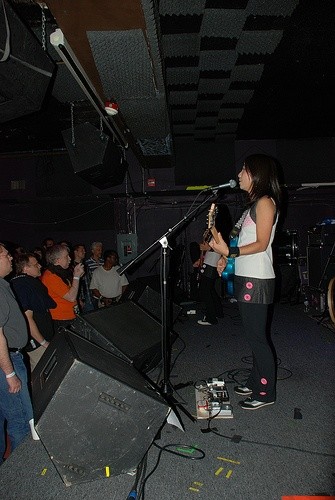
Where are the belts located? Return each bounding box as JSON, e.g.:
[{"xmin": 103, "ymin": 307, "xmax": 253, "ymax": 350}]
[{"xmin": 8, "ymin": 348, "xmax": 20, "ymax": 352}]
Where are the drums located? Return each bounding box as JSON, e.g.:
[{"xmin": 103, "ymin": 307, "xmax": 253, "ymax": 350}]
[{"xmin": 286, "ymin": 226, "xmax": 305, "ymax": 265}]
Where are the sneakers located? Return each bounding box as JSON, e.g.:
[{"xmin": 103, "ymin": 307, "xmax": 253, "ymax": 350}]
[
  {"xmin": 234, "ymin": 387, "xmax": 253, "ymax": 395},
  {"xmin": 197, "ymin": 319, "xmax": 212, "ymax": 326},
  {"xmin": 238, "ymin": 398, "xmax": 274, "ymax": 410}
]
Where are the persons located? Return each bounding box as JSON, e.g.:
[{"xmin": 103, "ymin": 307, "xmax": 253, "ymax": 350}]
[
  {"xmin": 89, "ymin": 250, "xmax": 129, "ymax": 309},
  {"xmin": 30, "ymin": 246, "xmax": 45, "ymax": 263},
  {"xmin": 39, "ymin": 245, "xmax": 85, "ymax": 331},
  {"xmin": 209, "ymin": 154, "xmax": 280, "ymax": 410},
  {"xmin": 0, "ymin": 243, "xmax": 34, "ymax": 463},
  {"xmin": 85, "ymin": 241, "xmax": 104, "ymax": 275},
  {"xmin": 11, "ymin": 254, "xmax": 57, "ymax": 371},
  {"xmin": 197, "ymin": 228, "xmax": 222, "ymax": 325},
  {"xmin": 71, "ymin": 243, "xmax": 91, "ymax": 314},
  {"xmin": 43, "ymin": 239, "xmax": 55, "ymax": 250}
]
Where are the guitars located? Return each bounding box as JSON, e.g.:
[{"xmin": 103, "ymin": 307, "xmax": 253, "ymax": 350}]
[
  {"xmin": 207, "ymin": 203, "xmax": 238, "ymax": 297},
  {"xmin": 198, "ymin": 231, "xmax": 210, "ymax": 268}
]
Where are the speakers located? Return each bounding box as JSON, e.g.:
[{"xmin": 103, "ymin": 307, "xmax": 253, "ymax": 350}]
[
  {"xmin": 69, "ymin": 300, "xmax": 172, "ymax": 362},
  {"xmin": 28, "ymin": 329, "xmax": 171, "ymax": 488},
  {"xmin": 136, "ymin": 283, "xmax": 183, "ymax": 328}
]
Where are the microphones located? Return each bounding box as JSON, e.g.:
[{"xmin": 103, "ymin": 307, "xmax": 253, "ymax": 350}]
[{"xmin": 204, "ymin": 180, "xmax": 236, "ymax": 191}]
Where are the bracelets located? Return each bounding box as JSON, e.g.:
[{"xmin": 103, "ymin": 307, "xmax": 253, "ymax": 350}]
[
  {"xmin": 226, "ymin": 247, "xmax": 239, "ymax": 258},
  {"xmin": 73, "ymin": 277, "xmax": 80, "ymax": 280},
  {"xmin": 5, "ymin": 371, "xmax": 15, "ymax": 378},
  {"xmin": 40, "ymin": 339, "xmax": 46, "ymax": 345}
]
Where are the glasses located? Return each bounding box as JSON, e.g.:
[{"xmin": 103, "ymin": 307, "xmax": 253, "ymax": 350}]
[{"xmin": 1, "ymin": 253, "xmax": 12, "ymax": 258}]
